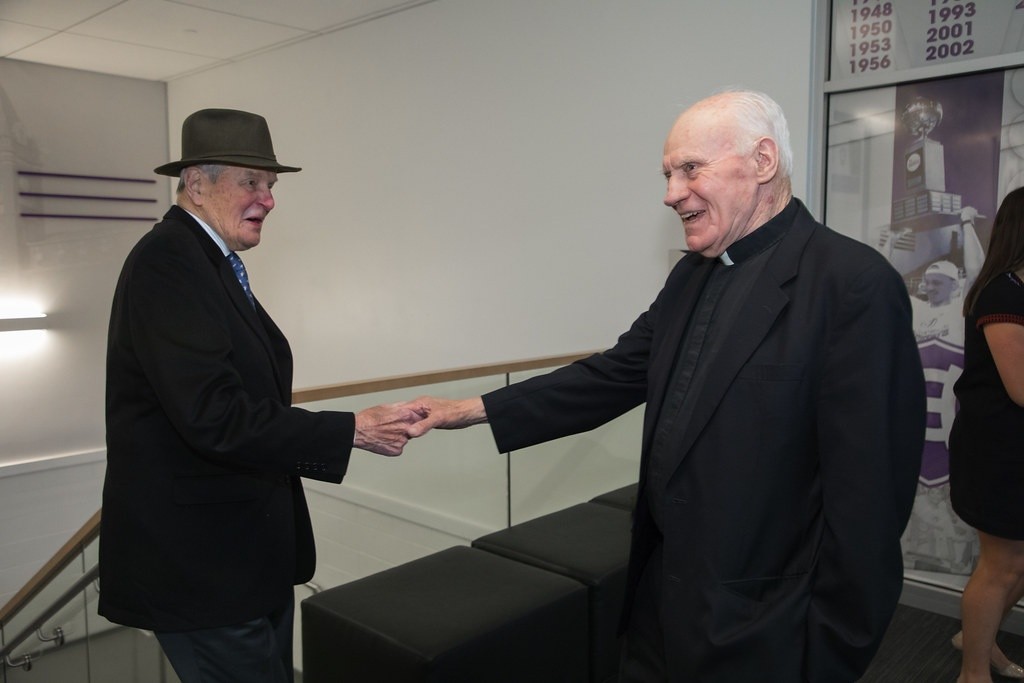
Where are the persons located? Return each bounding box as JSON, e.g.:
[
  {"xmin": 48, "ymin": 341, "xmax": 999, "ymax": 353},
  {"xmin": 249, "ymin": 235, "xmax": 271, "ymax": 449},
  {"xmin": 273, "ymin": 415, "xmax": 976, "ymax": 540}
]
[
  {"xmin": 95, "ymin": 108, "xmax": 424, "ymax": 683},
  {"xmin": 400, "ymin": 90, "xmax": 928, "ymax": 683},
  {"xmin": 949, "ymin": 186, "xmax": 1024, "ymax": 682}
]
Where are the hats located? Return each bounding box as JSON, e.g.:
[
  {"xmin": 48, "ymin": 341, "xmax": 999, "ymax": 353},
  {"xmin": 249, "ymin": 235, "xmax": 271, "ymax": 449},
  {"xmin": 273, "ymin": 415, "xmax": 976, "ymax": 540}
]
[
  {"xmin": 153, "ymin": 108, "xmax": 302, "ymax": 176},
  {"xmin": 926, "ymin": 261, "xmax": 958, "ymax": 281}
]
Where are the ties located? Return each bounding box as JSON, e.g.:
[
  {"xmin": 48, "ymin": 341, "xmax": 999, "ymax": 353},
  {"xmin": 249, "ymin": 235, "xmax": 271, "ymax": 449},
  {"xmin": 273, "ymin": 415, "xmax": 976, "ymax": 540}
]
[{"xmin": 226, "ymin": 251, "xmax": 257, "ymax": 313}]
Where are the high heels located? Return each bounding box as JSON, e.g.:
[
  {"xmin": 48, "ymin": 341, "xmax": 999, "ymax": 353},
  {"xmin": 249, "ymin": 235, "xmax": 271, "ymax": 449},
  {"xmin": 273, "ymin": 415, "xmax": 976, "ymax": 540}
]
[{"xmin": 952, "ymin": 631, "xmax": 1024, "ymax": 678}]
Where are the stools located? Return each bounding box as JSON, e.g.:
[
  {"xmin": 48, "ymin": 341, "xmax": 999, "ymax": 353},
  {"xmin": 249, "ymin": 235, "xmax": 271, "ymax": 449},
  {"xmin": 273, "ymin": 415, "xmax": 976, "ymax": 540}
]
[{"xmin": 300, "ymin": 481, "xmax": 639, "ymax": 683}]
